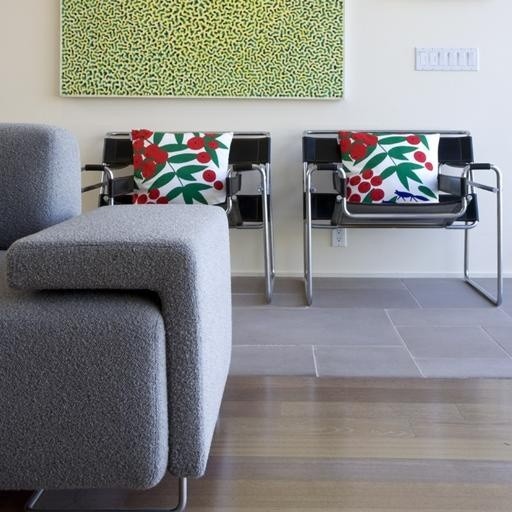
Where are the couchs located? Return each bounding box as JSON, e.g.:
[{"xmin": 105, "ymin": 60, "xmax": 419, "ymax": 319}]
[{"xmin": 0, "ymin": 123, "xmax": 232, "ymax": 512}]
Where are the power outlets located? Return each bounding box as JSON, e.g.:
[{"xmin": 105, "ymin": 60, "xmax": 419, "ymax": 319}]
[{"xmin": 331, "ymin": 228, "xmax": 346, "ymax": 248}]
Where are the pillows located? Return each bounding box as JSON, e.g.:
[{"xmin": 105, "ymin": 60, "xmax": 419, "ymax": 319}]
[
  {"xmin": 129, "ymin": 129, "xmax": 234, "ymax": 205},
  {"xmin": 336, "ymin": 129, "xmax": 439, "ymax": 204}
]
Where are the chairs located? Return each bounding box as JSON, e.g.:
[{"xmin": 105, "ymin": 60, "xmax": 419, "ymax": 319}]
[
  {"xmin": 80, "ymin": 130, "xmax": 275, "ymax": 302},
  {"xmin": 302, "ymin": 129, "xmax": 502, "ymax": 307}
]
